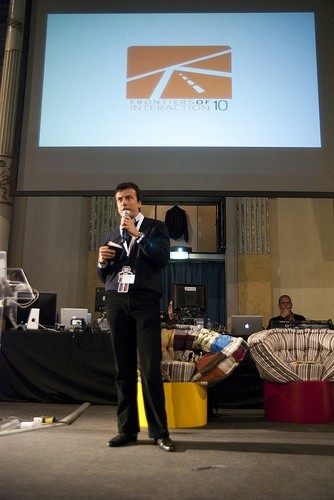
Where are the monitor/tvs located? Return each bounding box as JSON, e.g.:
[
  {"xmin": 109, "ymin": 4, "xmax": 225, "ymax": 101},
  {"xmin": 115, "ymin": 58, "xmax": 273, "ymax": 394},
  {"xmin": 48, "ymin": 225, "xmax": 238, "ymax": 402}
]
[
  {"xmin": 17, "ymin": 291, "xmax": 57, "ymax": 330},
  {"xmin": 95, "ymin": 287, "xmax": 107, "ymax": 312},
  {"xmin": 171, "ymin": 282, "xmax": 208, "ymax": 314}
]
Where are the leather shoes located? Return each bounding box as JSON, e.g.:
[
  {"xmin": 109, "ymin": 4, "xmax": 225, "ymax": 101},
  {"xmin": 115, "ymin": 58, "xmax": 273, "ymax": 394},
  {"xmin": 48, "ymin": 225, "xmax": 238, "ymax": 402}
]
[
  {"xmin": 154, "ymin": 436, "xmax": 176, "ymax": 451},
  {"xmin": 109, "ymin": 433, "xmax": 138, "ymax": 447}
]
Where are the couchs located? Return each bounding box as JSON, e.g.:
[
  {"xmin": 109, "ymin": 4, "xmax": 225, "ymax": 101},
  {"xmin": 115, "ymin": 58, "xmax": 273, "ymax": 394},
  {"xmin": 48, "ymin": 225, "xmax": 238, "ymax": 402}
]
[
  {"xmin": 247, "ymin": 327, "xmax": 334, "ymax": 424},
  {"xmin": 136, "ymin": 325, "xmax": 250, "ymax": 429}
]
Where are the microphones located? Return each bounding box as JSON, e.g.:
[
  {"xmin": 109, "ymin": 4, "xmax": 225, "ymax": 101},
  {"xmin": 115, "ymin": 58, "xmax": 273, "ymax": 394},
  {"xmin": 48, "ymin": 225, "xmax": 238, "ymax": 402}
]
[{"xmin": 121, "ymin": 210, "xmax": 130, "ymax": 243}]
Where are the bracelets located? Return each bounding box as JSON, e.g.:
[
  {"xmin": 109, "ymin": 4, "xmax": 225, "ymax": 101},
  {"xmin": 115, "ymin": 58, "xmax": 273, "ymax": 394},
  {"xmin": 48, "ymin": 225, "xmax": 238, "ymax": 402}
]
[{"xmin": 136, "ymin": 232, "xmax": 144, "ymax": 241}]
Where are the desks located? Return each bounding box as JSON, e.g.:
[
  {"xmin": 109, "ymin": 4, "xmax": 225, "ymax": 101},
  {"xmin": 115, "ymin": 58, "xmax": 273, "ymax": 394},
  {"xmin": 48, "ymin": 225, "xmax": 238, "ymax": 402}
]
[{"xmin": 0, "ymin": 330, "xmax": 264, "ymax": 411}]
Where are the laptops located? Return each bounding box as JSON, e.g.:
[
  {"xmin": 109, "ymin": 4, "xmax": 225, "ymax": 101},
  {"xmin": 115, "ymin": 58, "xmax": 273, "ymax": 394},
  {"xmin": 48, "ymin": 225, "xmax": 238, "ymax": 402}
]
[
  {"xmin": 61, "ymin": 308, "xmax": 88, "ymax": 329},
  {"xmin": 230, "ymin": 315, "xmax": 264, "ymax": 337}
]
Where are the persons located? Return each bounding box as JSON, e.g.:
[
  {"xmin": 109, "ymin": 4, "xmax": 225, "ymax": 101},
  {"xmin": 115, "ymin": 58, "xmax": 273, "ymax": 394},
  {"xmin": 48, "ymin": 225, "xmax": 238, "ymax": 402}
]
[
  {"xmin": 97, "ymin": 181, "xmax": 176, "ymax": 452},
  {"xmin": 266, "ymin": 295, "xmax": 307, "ymax": 330}
]
